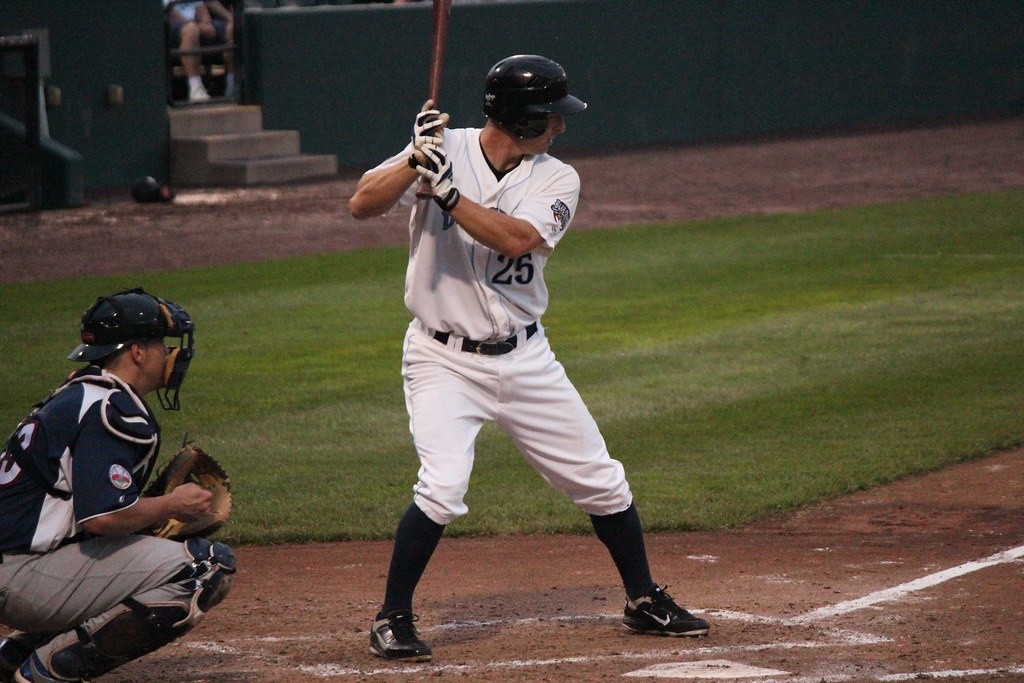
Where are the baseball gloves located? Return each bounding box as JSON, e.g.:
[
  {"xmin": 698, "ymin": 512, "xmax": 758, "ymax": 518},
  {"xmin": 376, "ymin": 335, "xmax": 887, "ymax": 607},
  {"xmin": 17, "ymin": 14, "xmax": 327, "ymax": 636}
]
[{"xmin": 142, "ymin": 431, "xmax": 231, "ymax": 540}]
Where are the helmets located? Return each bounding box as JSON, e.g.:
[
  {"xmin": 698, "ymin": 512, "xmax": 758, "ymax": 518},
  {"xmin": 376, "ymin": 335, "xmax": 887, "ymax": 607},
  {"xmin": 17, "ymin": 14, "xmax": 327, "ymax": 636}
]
[
  {"xmin": 66, "ymin": 288, "xmax": 165, "ymax": 360},
  {"xmin": 483, "ymin": 56, "xmax": 587, "ymax": 139}
]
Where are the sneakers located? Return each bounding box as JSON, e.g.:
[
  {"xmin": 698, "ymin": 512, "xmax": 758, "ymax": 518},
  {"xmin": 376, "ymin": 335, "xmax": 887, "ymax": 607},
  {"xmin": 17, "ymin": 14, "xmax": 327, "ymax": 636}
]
[
  {"xmin": 369, "ymin": 609, "xmax": 432, "ymax": 663},
  {"xmin": 622, "ymin": 582, "xmax": 710, "ymax": 635},
  {"xmin": 14, "ymin": 649, "xmax": 87, "ymax": 683},
  {"xmin": 0, "ymin": 637, "xmax": 32, "ymax": 672}
]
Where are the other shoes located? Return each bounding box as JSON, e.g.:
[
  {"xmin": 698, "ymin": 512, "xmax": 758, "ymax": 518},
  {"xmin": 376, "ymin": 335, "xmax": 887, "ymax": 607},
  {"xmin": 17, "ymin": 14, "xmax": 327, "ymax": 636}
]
[{"xmin": 191, "ymin": 86, "xmax": 212, "ymax": 102}]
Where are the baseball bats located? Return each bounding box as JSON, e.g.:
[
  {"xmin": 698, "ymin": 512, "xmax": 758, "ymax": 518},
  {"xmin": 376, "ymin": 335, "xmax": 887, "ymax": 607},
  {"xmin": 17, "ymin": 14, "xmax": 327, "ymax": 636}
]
[{"xmin": 418, "ymin": 0, "xmax": 452, "ymax": 199}]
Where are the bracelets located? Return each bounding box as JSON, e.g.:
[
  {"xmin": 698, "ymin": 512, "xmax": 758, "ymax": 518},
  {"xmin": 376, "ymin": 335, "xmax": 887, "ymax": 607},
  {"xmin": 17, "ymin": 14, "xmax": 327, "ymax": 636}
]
[{"xmin": 203, "ymin": 19, "xmax": 214, "ymax": 24}]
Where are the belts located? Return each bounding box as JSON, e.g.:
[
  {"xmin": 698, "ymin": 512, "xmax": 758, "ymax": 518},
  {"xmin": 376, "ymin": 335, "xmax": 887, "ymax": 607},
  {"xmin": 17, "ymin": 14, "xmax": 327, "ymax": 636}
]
[{"xmin": 434, "ymin": 321, "xmax": 538, "ymax": 357}]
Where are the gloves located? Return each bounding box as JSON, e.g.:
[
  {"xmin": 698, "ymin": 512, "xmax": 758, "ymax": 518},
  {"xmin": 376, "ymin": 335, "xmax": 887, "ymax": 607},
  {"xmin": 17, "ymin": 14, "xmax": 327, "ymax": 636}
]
[
  {"xmin": 410, "ymin": 140, "xmax": 460, "ymax": 213},
  {"xmin": 407, "ymin": 99, "xmax": 450, "ymax": 169}
]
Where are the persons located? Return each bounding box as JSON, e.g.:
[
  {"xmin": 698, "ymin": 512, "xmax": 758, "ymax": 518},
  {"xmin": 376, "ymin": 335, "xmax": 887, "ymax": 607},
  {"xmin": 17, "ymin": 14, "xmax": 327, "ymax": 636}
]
[
  {"xmin": 161, "ymin": 0, "xmax": 235, "ymax": 103},
  {"xmin": 350, "ymin": 55, "xmax": 711, "ymax": 661},
  {"xmin": 0, "ymin": 288, "xmax": 237, "ymax": 683}
]
